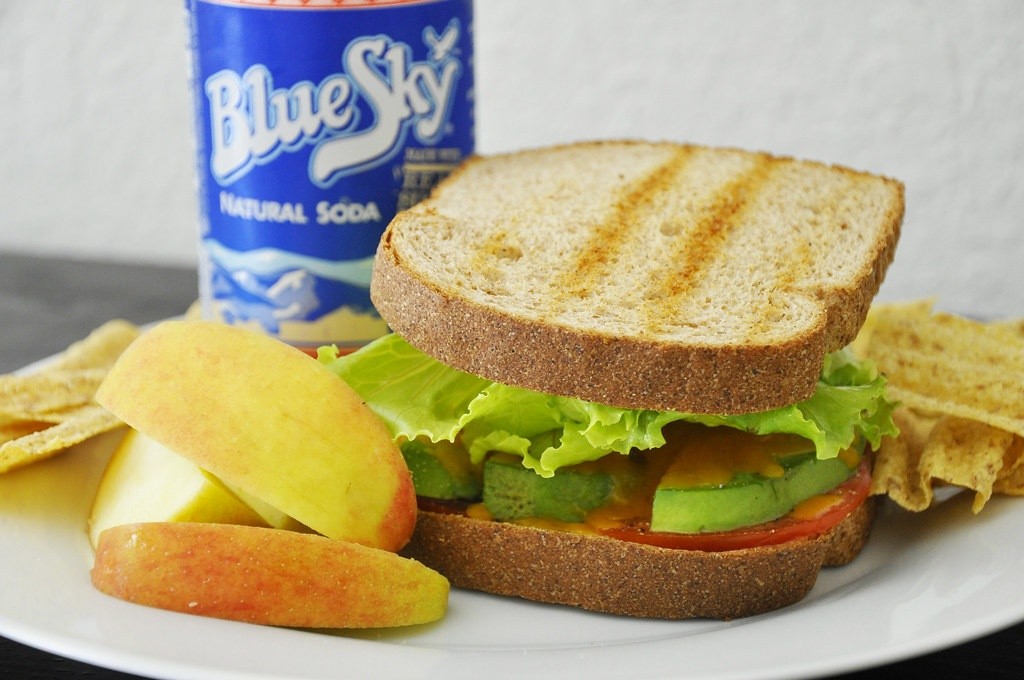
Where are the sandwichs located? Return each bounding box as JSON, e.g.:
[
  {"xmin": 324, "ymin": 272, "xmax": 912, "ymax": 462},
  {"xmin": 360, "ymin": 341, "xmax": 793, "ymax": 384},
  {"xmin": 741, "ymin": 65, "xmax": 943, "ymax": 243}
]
[{"xmin": 306, "ymin": 133, "xmax": 909, "ymax": 622}]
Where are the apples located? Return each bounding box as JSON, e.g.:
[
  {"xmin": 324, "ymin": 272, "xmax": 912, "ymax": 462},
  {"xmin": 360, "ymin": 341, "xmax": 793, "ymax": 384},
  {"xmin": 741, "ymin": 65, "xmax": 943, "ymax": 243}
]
[{"xmin": 88, "ymin": 320, "xmax": 450, "ymax": 628}]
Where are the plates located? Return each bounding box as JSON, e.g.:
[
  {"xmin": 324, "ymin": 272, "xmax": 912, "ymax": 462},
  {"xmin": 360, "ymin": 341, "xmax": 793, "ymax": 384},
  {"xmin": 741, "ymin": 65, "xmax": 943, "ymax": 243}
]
[{"xmin": 8, "ymin": 319, "xmax": 1024, "ymax": 680}]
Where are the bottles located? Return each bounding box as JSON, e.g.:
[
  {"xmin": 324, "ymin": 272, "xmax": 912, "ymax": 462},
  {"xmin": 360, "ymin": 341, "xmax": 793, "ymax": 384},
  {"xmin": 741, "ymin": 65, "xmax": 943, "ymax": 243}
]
[{"xmin": 188, "ymin": 1, "xmax": 482, "ymax": 355}]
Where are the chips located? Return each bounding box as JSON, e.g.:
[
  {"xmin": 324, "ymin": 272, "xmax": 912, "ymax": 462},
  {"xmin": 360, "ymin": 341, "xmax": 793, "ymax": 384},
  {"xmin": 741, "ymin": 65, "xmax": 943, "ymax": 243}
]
[
  {"xmin": 848, "ymin": 299, "xmax": 1024, "ymax": 515},
  {"xmin": 1, "ymin": 315, "xmax": 140, "ymax": 476}
]
